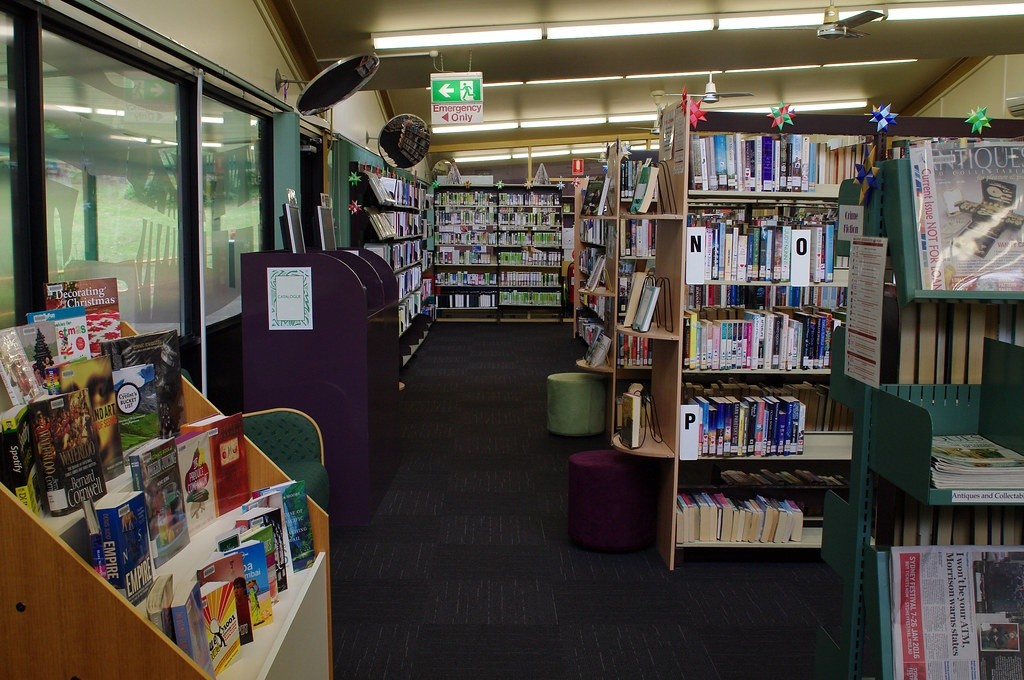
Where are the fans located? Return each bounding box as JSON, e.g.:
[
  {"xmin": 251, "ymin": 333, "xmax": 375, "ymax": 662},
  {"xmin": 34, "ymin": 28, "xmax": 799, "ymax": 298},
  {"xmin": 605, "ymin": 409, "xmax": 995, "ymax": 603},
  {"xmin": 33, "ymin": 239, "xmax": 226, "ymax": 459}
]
[
  {"xmin": 653, "ymin": 73, "xmax": 754, "ymax": 104},
  {"xmin": 625, "ymin": 90, "xmax": 666, "ymax": 135},
  {"xmin": 758, "ymin": 0, "xmax": 885, "ymax": 41}
]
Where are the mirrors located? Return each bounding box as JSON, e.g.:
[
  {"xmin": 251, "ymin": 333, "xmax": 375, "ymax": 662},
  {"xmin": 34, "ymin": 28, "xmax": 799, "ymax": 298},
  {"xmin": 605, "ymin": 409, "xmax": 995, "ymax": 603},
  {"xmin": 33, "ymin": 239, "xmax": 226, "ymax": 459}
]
[
  {"xmin": 275, "ymin": 51, "xmax": 380, "ymax": 116},
  {"xmin": 366, "ymin": 113, "xmax": 431, "ymax": 168}
]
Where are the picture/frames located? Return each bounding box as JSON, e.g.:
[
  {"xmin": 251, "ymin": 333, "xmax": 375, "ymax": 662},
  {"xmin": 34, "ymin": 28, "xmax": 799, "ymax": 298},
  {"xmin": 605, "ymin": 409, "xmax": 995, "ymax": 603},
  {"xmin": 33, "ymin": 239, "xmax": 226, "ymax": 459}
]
[
  {"xmin": 318, "ymin": 206, "xmax": 337, "ymax": 251},
  {"xmin": 286, "ymin": 203, "xmax": 306, "ymax": 254}
]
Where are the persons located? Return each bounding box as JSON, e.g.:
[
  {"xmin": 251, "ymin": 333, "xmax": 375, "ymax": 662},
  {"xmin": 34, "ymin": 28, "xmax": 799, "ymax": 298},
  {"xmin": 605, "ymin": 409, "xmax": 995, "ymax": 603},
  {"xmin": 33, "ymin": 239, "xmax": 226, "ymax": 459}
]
[{"xmin": 232, "ymin": 576, "xmax": 249, "ymax": 626}]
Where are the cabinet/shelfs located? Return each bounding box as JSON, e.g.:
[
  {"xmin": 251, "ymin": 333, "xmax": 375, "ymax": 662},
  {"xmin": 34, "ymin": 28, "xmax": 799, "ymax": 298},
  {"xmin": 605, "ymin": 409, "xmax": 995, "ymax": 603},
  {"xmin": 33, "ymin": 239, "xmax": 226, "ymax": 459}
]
[
  {"xmin": 384, "ymin": 116, "xmax": 430, "ymax": 164},
  {"xmin": 563, "ymin": 95, "xmax": 1024, "ymax": 680},
  {"xmin": 434, "ymin": 185, "xmax": 564, "ymax": 323},
  {"xmin": 348, "ymin": 161, "xmax": 436, "ymax": 371}
]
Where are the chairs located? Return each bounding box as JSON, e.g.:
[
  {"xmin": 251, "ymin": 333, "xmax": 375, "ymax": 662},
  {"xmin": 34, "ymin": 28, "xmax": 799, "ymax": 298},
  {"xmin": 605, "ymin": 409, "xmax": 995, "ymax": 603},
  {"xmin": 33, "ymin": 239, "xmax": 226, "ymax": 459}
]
[{"xmin": 180, "ymin": 368, "xmax": 331, "ymax": 509}]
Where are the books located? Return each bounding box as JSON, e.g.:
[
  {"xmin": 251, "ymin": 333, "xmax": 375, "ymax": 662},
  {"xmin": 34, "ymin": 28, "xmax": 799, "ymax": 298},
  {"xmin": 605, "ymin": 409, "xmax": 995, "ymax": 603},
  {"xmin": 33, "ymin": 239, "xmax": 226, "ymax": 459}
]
[
  {"xmin": 0, "ymin": 276, "xmax": 316, "ymax": 680},
  {"xmin": 367, "ymin": 132, "xmax": 864, "ymax": 542},
  {"xmin": 931, "ymin": 434, "xmax": 1024, "ymax": 489}
]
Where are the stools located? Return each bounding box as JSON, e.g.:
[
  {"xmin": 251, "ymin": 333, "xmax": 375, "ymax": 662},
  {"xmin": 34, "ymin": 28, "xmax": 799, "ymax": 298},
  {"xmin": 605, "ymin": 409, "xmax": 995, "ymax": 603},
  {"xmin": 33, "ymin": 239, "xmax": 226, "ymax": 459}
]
[
  {"xmin": 546, "ymin": 372, "xmax": 606, "ymax": 437},
  {"xmin": 569, "ymin": 450, "xmax": 658, "ymax": 552}
]
